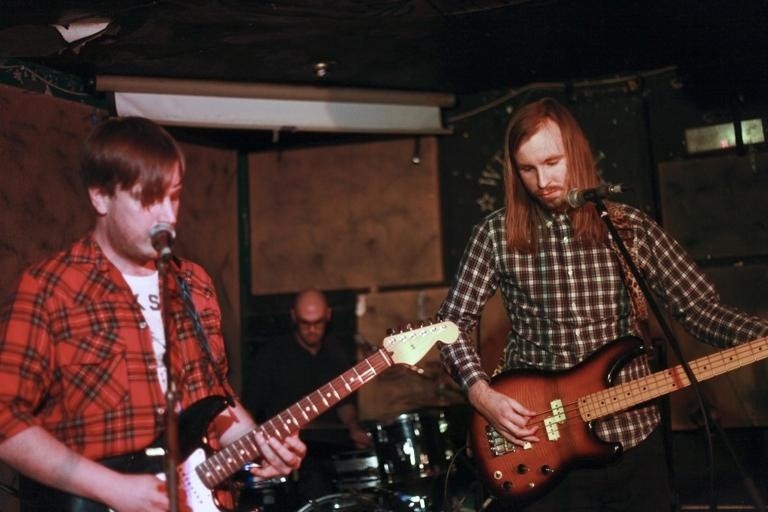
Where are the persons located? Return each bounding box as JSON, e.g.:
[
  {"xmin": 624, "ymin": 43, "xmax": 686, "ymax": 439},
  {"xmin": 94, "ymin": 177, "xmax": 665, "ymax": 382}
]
[
  {"xmin": 433, "ymin": 97, "xmax": 768, "ymax": 511},
  {"xmin": 246, "ymin": 285, "xmax": 373, "ymax": 450},
  {"xmin": 1, "ymin": 115, "xmax": 307, "ymax": 512}
]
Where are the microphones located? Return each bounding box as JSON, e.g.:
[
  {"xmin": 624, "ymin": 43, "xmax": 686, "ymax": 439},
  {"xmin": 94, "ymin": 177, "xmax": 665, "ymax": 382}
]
[
  {"xmin": 149, "ymin": 222, "xmax": 177, "ymax": 264},
  {"xmin": 565, "ymin": 183, "xmax": 624, "ymax": 209}
]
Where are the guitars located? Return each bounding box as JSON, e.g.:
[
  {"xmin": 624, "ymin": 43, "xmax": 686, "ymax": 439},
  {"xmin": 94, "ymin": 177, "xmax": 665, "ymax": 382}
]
[
  {"xmin": 470, "ymin": 334, "xmax": 768, "ymax": 494},
  {"xmin": 106, "ymin": 318, "xmax": 462, "ymax": 512}
]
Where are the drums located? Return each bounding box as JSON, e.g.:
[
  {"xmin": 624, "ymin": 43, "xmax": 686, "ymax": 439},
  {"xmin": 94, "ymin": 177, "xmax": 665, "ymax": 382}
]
[
  {"xmin": 370, "ymin": 409, "xmax": 438, "ymax": 485},
  {"xmin": 332, "ymin": 454, "xmax": 386, "ymax": 488}
]
